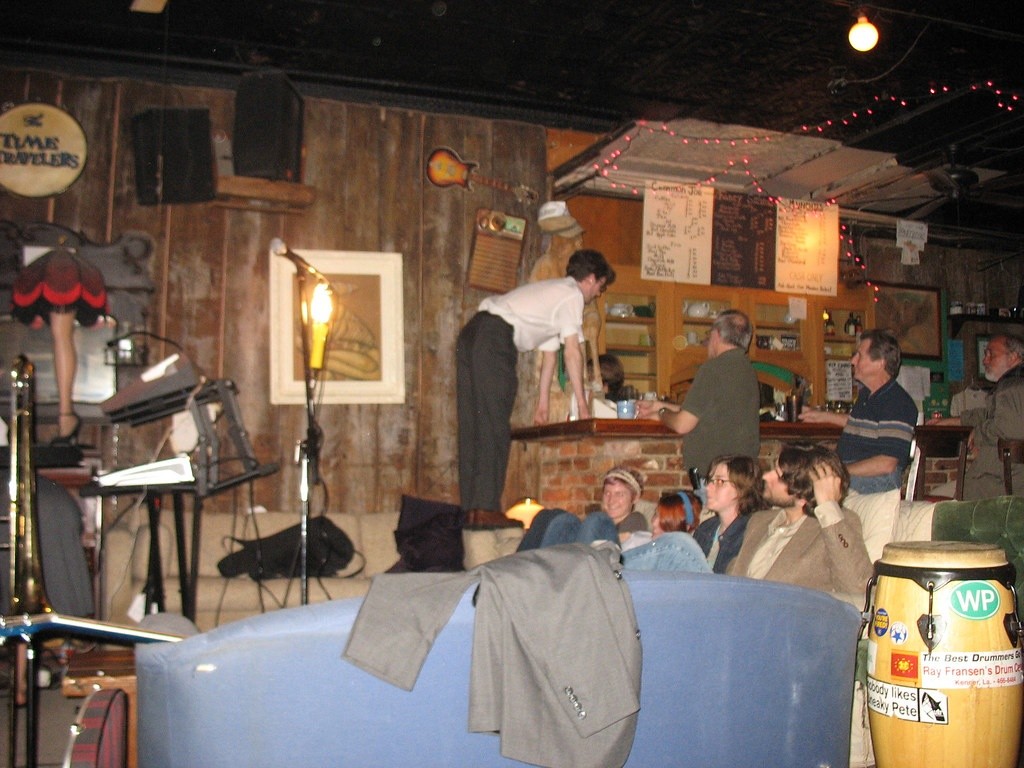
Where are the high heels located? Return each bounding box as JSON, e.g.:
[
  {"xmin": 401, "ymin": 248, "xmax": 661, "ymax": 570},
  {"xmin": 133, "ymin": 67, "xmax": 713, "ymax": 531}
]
[{"xmin": 49, "ymin": 412, "xmax": 82, "ymax": 447}]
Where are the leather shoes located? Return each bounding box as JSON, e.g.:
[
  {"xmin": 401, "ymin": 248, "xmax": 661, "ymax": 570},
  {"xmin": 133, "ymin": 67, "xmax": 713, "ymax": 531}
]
[{"xmin": 465, "ymin": 510, "xmax": 525, "ymax": 531}]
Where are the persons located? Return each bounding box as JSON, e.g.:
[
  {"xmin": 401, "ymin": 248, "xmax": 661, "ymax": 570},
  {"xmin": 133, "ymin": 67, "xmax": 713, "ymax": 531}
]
[
  {"xmin": 782, "ymin": 328, "xmax": 920, "ymax": 566},
  {"xmin": 13, "ymin": 247, "xmax": 107, "ymax": 448},
  {"xmin": 586, "ymin": 354, "xmax": 625, "ymax": 404},
  {"xmin": 635, "ymin": 309, "xmax": 760, "ymax": 502},
  {"xmin": 491, "ymin": 437, "xmax": 873, "ymax": 600},
  {"xmin": 923, "ymin": 334, "xmax": 1024, "ymax": 501},
  {"xmin": 455, "ymin": 249, "xmax": 611, "ymax": 531}
]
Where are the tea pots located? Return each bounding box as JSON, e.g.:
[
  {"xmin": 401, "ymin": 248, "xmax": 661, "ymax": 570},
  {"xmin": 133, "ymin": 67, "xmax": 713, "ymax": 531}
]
[
  {"xmin": 618, "ymin": 385, "xmax": 640, "ymax": 400},
  {"xmin": 683, "ymin": 301, "xmax": 710, "ymax": 317},
  {"xmin": 607, "ymin": 303, "xmax": 633, "ymax": 317}
]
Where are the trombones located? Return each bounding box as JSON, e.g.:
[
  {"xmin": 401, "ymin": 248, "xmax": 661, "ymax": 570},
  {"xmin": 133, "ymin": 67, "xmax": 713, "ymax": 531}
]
[{"xmin": 9, "ymin": 353, "xmax": 58, "ymax": 768}]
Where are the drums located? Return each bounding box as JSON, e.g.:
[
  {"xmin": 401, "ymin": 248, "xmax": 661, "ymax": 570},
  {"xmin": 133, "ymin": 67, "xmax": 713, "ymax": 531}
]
[{"xmin": 866, "ymin": 541, "xmax": 1024, "ymax": 768}]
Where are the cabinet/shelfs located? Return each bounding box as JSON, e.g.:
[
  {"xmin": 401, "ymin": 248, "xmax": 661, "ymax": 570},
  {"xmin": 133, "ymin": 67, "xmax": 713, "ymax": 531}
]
[{"xmin": 585, "ymin": 279, "xmax": 863, "ymax": 411}]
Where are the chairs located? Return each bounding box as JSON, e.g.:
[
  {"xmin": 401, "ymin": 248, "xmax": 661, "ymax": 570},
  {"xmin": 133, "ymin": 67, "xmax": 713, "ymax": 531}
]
[
  {"xmin": 997, "ymin": 437, "xmax": 1024, "ymax": 495},
  {"xmin": 900, "ymin": 439, "xmax": 969, "ymax": 502}
]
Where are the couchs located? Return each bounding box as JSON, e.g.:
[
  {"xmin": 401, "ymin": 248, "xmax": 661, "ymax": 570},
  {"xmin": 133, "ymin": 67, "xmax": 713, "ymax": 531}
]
[{"xmin": 101, "ymin": 487, "xmax": 1024, "ymax": 768}]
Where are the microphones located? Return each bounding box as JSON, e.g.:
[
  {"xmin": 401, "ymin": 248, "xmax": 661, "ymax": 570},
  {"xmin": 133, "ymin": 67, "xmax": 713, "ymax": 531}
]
[{"xmin": 271, "ymin": 238, "xmax": 319, "ymax": 278}]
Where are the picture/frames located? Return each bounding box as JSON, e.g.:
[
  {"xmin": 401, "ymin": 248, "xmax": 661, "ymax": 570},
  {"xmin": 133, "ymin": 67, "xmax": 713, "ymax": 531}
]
[
  {"xmin": 268, "ymin": 248, "xmax": 406, "ymax": 404},
  {"xmin": 864, "ymin": 279, "xmax": 943, "ymax": 362}
]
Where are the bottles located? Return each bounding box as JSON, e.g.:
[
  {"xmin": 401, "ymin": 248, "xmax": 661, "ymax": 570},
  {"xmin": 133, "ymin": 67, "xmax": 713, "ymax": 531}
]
[
  {"xmin": 756, "ymin": 334, "xmax": 800, "ymax": 352},
  {"xmin": 823, "ymin": 306, "xmax": 835, "ymax": 336},
  {"xmin": 844, "ymin": 311, "xmax": 863, "ymax": 348},
  {"xmin": 815, "ymin": 400, "xmax": 855, "ymax": 414},
  {"xmin": 702, "ymin": 331, "xmax": 711, "ymax": 345},
  {"xmin": 949, "ymin": 300, "xmax": 1024, "ymax": 319},
  {"xmin": 104, "ymin": 321, "xmax": 146, "ymax": 365},
  {"xmin": 786, "ymin": 377, "xmax": 803, "ymax": 423}
]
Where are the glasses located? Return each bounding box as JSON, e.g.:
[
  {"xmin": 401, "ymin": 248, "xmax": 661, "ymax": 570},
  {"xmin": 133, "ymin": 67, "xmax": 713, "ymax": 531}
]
[
  {"xmin": 704, "ymin": 322, "xmax": 723, "ymax": 337},
  {"xmin": 595, "ymin": 276, "xmax": 607, "ymax": 293},
  {"xmin": 705, "ymin": 478, "xmax": 734, "ymax": 487}
]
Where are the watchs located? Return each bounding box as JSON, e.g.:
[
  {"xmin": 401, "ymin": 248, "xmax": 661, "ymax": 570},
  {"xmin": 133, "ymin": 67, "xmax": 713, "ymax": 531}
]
[{"xmin": 659, "ymin": 407, "xmax": 670, "ymax": 419}]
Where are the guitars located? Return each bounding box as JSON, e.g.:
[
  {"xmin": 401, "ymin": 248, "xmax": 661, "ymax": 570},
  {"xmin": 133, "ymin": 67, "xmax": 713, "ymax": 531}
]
[{"xmin": 424, "ymin": 146, "xmax": 540, "ymax": 205}]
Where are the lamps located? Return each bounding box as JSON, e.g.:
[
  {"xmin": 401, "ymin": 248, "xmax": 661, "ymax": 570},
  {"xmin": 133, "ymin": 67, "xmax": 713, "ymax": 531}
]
[{"xmin": 8, "ymin": 244, "xmax": 110, "ymax": 469}]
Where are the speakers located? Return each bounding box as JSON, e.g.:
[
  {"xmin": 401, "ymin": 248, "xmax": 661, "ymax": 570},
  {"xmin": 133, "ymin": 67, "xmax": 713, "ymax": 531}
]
[
  {"xmin": 130, "ymin": 105, "xmax": 216, "ymax": 206},
  {"xmin": 230, "ymin": 74, "xmax": 305, "ymax": 184},
  {"xmin": 218, "ymin": 515, "xmax": 355, "ymax": 579}
]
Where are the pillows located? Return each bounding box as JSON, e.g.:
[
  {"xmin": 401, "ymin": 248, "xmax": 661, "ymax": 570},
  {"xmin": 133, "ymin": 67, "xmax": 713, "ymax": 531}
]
[
  {"xmin": 393, "ymin": 512, "xmax": 466, "ymax": 573},
  {"xmin": 840, "ymin": 490, "xmax": 901, "ymax": 567}
]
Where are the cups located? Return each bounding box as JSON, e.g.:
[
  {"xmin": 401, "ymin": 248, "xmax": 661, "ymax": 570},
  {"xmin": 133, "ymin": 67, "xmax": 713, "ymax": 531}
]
[
  {"xmin": 775, "ymin": 403, "xmax": 785, "ymax": 417},
  {"xmin": 686, "ymin": 331, "xmax": 701, "ymax": 345},
  {"xmin": 616, "ymin": 399, "xmax": 640, "ymax": 419}
]
